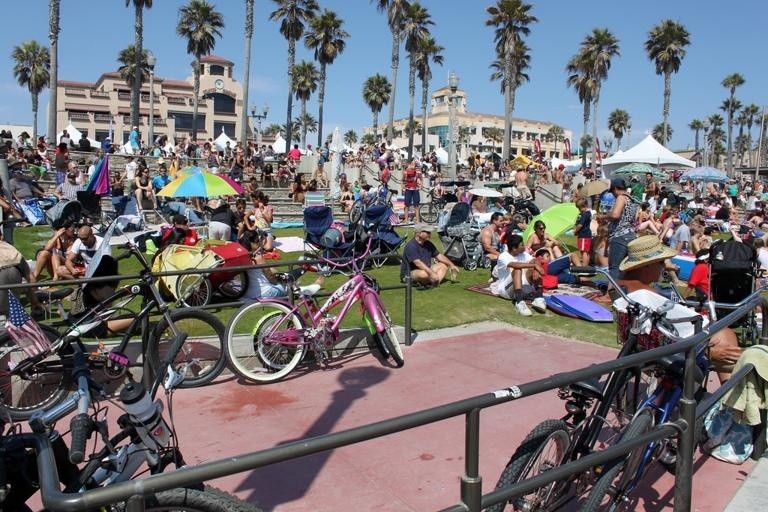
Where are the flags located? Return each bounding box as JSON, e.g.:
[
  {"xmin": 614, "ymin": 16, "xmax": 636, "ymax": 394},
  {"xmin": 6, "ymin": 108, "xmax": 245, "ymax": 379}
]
[{"xmin": 5, "ymin": 288, "xmax": 54, "ymax": 359}]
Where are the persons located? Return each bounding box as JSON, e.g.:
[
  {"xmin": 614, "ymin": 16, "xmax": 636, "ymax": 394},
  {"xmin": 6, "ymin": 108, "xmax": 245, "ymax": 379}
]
[
  {"xmin": 526, "ymin": 249, "xmax": 608, "ymax": 296},
  {"xmin": 287, "ymin": 144, "xmax": 302, "ymax": 161},
  {"xmin": 56, "ymin": 225, "xmax": 111, "ymax": 280},
  {"xmin": 591, "ymin": 178, "xmax": 641, "ymax": 305},
  {"xmin": 0, "ymin": 124, "xmax": 124, "ymax": 228},
  {"xmin": 288, "ymin": 172, "xmax": 310, "ymax": 203},
  {"xmin": 230, "ymin": 200, "xmax": 250, "ymax": 243},
  {"xmin": 304, "ymin": 144, "xmax": 313, "ymax": 157},
  {"xmin": 378, "ymin": 165, "xmax": 391, "ymax": 183},
  {"xmin": 635, "ymin": 175, "xmax": 766, "ymax": 237},
  {"xmin": 420, "ymin": 148, "xmax": 571, "ymax": 223},
  {"xmin": 152, "ymin": 167, "xmax": 174, "ymax": 210},
  {"xmin": 230, "ymin": 165, "xmax": 243, "ymax": 183},
  {"xmin": 128, "ymin": 125, "xmax": 142, "ymax": 156},
  {"xmin": 205, "ymin": 198, "xmax": 236, "ymax": 244},
  {"xmin": 526, "ymin": 220, "xmax": 563, "ymax": 263},
  {"xmin": 139, "ymin": 124, "xmax": 221, "ymax": 170},
  {"xmin": 131, "ymin": 168, "xmax": 158, "ymax": 210},
  {"xmin": 0, "ymin": 227, "xmax": 52, "ymax": 322},
  {"xmin": 473, "ymin": 212, "xmax": 505, "ymax": 261},
  {"xmin": 400, "ymin": 223, "xmax": 460, "ymax": 290},
  {"xmin": 133, "ymin": 157, "xmax": 147, "ymax": 190},
  {"xmin": 313, "ymin": 138, "xmax": 398, "ymax": 169},
  {"xmin": 311, "ymin": 162, "xmax": 328, "ymax": 189},
  {"xmin": 221, "ymin": 127, "xmax": 278, "ymax": 164},
  {"xmin": 277, "ymin": 161, "xmax": 293, "ymax": 181},
  {"xmin": 359, "ymin": 184, "xmax": 373, "ymax": 206},
  {"xmin": 30, "ymin": 218, "xmax": 76, "ymax": 282},
  {"xmin": 571, "ymin": 161, "xmax": 592, "ymax": 203},
  {"xmin": 399, "ymin": 161, "xmax": 424, "ymax": 225},
  {"xmin": 238, "ymin": 230, "xmax": 326, "ymax": 300},
  {"xmin": 150, "ymin": 214, "xmax": 198, "ymax": 266},
  {"xmin": 67, "ymin": 253, "xmax": 142, "ymax": 338},
  {"xmin": 506, "ymin": 214, "xmax": 527, "ymax": 240},
  {"xmin": 351, "ymin": 180, "xmax": 361, "ymax": 193},
  {"xmin": 219, "ymin": 160, "xmax": 232, "ymax": 178},
  {"xmin": 243, "ymin": 176, "xmax": 260, "ymax": 200},
  {"xmin": 119, "ymin": 153, "xmax": 139, "ymax": 198},
  {"xmin": 489, "ymin": 234, "xmax": 548, "ymax": 318},
  {"xmin": 279, "ymin": 153, "xmax": 298, "ymax": 177},
  {"xmin": 340, "ymin": 182, "xmax": 357, "ymax": 215},
  {"xmin": 498, "ymin": 213, "xmax": 514, "ymax": 255},
  {"xmin": 592, "ymin": 235, "xmax": 760, "ymax": 458},
  {"xmin": 261, "ymin": 164, "xmax": 278, "ymax": 188},
  {"xmin": 572, "ymin": 198, "xmax": 592, "ymax": 267},
  {"xmin": 313, "ymin": 172, "xmax": 327, "ymax": 189},
  {"xmin": 244, "ymin": 161, "xmax": 256, "ymax": 178},
  {"xmin": 339, "ymin": 173, "xmax": 349, "ymax": 213},
  {"xmin": 243, "ymin": 189, "xmax": 277, "ymax": 251}
]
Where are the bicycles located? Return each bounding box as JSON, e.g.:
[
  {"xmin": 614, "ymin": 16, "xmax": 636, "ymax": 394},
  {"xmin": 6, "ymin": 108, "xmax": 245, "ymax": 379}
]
[
  {"xmin": 349, "ymin": 188, "xmax": 398, "ymax": 223},
  {"xmin": 420, "ymin": 188, "xmax": 446, "ymax": 222}
]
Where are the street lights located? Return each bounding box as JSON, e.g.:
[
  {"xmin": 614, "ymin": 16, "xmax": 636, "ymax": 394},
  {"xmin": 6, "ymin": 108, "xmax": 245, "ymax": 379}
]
[
  {"xmin": 251, "ymin": 100, "xmax": 269, "ymax": 157},
  {"xmin": 699, "ymin": 116, "xmax": 712, "ymax": 187},
  {"xmin": 442, "ymin": 70, "xmax": 463, "ymax": 182}
]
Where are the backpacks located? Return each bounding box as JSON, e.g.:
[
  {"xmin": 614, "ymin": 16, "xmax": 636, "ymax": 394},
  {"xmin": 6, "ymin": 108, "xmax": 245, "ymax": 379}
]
[
  {"xmin": 70, "ymin": 287, "xmax": 85, "ymax": 316},
  {"xmin": 406, "ymin": 170, "xmax": 417, "ymax": 188}
]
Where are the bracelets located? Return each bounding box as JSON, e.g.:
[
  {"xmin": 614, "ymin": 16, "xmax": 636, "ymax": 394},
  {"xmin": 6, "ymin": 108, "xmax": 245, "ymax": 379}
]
[{"xmin": 262, "ymin": 211, "xmax": 265, "ymax": 215}]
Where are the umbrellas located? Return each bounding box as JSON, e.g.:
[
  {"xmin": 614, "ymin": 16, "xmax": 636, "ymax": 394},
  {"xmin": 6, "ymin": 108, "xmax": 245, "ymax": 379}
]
[
  {"xmin": 462, "ymin": 186, "xmax": 505, "ymax": 207},
  {"xmin": 154, "ymin": 171, "xmax": 246, "ymax": 198},
  {"xmin": 522, "ymin": 202, "xmax": 582, "ymax": 255},
  {"xmin": 608, "ymin": 161, "xmax": 670, "ymax": 182},
  {"xmin": 674, "ymin": 165, "xmax": 733, "ymax": 185},
  {"xmin": 174, "ymin": 166, "xmax": 207, "ymax": 179},
  {"xmin": 573, "ymin": 178, "xmax": 613, "ymax": 212}
]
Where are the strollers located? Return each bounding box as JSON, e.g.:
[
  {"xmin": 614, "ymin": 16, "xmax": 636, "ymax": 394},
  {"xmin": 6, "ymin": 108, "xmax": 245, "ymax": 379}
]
[
  {"xmin": 704, "ymin": 239, "xmax": 760, "ymax": 346},
  {"xmin": 438, "ymin": 203, "xmax": 490, "ymax": 270},
  {"xmin": 35, "ymin": 194, "xmax": 84, "ymax": 265},
  {"xmin": 154, "ymin": 234, "xmax": 251, "ymax": 307}
]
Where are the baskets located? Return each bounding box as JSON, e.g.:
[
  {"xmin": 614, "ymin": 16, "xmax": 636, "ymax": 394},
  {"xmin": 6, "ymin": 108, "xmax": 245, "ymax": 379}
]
[{"xmin": 612, "ymin": 305, "xmax": 678, "ymax": 351}]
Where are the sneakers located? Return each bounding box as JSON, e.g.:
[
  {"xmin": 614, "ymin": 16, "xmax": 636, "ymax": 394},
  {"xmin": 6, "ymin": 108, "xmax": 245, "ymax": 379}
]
[
  {"xmin": 515, "ymin": 301, "xmax": 533, "ymax": 317},
  {"xmin": 531, "ymin": 297, "xmax": 547, "ymax": 313}
]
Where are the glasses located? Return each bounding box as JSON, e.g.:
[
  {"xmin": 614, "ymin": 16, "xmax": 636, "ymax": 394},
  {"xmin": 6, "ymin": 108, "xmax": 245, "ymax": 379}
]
[
  {"xmin": 78, "ymin": 235, "xmax": 90, "ymax": 240},
  {"xmin": 238, "ymin": 206, "xmax": 246, "ymax": 210},
  {"xmin": 73, "ymin": 166, "xmax": 78, "ymax": 169}
]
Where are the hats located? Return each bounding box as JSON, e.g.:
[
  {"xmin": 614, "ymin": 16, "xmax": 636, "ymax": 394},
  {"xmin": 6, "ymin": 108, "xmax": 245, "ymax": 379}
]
[
  {"xmin": 68, "ymin": 172, "xmax": 77, "ymax": 178},
  {"xmin": 7, "ymin": 156, "xmax": 22, "ymax": 167},
  {"xmin": 559, "ymin": 164, "xmax": 566, "ymax": 169},
  {"xmin": 619, "ymin": 235, "xmax": 677, "ymax": 272},
  {"xmin": 124, "ymin": 153, "xmax": 134, "ymax": 159},
  {"xmin": 170, "ymin": 214, "xmax": 187, "ymax": 224},
  {"xmin": 158, "ymin": 157, "xmax": 166, "ymax": 164},
  {"xmin": 414, "ymin": 223, "xmax": 434, "ymax": 233},
  {"xmin": 608, "ymin": 178, "xmax": 624, "ymax": 193},
  {"xmin": 513, "ymin": 213, "xmax": 527, "ymax": 223}
]
